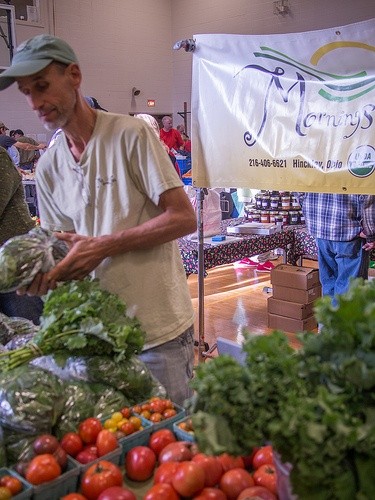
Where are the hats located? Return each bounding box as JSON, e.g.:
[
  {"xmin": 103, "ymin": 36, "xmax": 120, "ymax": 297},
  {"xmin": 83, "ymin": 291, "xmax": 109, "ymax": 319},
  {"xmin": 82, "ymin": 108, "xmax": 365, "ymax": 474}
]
[
  {"xmin": 12, "ymin": 129, "xmax": 23, "ymax": 136},
  {"xmin": 0, "ymin": 123, "xmax": 9, "ymax": 130},
  {"xmin": 1, "ymin": 33, "xmax": 79, "ymax": 91}
]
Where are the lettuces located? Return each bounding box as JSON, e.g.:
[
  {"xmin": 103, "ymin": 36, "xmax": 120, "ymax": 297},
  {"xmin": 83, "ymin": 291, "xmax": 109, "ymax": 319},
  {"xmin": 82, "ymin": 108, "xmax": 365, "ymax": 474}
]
[{"xmin": 185, "ymin": 276, "xmax": 375, "ymax": 500}]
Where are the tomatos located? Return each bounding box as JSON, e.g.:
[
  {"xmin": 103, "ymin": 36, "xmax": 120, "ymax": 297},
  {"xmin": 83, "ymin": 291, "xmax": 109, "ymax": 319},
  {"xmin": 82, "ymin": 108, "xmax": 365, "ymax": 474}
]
[{"xmin": 0, "ymin": 396, "xmax": 278, "ymax": 500}]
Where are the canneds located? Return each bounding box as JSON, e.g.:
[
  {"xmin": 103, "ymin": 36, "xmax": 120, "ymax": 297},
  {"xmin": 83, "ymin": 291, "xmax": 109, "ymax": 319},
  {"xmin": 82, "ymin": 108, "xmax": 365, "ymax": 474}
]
[{"xmin": 243, "ymin": 190, "xmax": 306, "ymax": 225}]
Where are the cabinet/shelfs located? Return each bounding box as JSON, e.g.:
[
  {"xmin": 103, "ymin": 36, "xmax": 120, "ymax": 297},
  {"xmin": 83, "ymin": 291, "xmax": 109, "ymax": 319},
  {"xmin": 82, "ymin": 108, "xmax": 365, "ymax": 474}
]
[{"xmin": 22, "ymin": 180, "xmax": 35, "ymax": 217}]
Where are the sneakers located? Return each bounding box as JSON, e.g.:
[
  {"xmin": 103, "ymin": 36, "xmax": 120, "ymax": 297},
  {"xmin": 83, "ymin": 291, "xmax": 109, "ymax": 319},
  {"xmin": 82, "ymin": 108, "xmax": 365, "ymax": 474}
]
[
  {"xmin": 256, "ymin": 260, "xmax": 275, "ymax": 272},
  {"xmin": 238, "ymin": 258, "xmax": 259, "ymax": 266}
]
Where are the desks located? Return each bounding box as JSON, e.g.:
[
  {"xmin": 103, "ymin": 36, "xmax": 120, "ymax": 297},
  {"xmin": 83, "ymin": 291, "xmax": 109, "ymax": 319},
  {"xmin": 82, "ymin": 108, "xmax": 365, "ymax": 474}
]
[{"xmin": 177, "ymin": 218, "xmax": 318, "ymax": 278}]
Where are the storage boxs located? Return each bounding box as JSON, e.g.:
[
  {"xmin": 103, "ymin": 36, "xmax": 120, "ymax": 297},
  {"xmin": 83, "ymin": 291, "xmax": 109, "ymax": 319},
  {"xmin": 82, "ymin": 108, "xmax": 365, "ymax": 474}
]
[
  {"xmin": 0, "ymin": 397, "xmax": 198, "ymax": 500},
  {"xmin": 177, "ymin": 159, "xmax": 192, "ymax": 185},
  {"xmin": 266, "ymin": 263, "xmax": 321, "ymax": 333}
]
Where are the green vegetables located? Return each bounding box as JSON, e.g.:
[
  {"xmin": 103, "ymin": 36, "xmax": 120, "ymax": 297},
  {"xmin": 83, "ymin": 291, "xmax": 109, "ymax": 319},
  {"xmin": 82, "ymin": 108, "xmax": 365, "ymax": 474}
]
[{"xmin": 0, "ymin": 228, "xmax": 157, "ymax": 467}]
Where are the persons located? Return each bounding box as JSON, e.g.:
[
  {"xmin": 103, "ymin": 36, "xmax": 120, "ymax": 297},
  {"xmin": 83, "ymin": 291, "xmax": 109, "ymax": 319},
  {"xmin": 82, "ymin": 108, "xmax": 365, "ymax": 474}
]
[
  {"xmin": 0, "ymin": 34, "xmax": 197, "ymax": 407},
  {"xmin": 292, "ymin": 192, "xmax": 375, "ymax": 333},
  {"xmin": 0, "ymin": 96, "xmax": 279, "ymax": 324}
]
